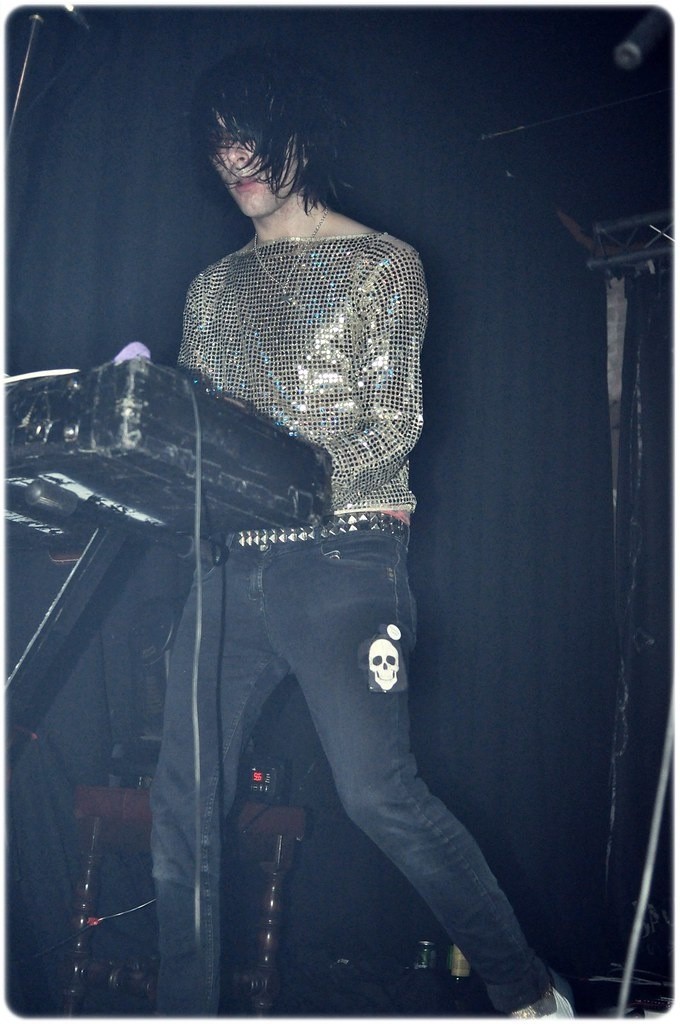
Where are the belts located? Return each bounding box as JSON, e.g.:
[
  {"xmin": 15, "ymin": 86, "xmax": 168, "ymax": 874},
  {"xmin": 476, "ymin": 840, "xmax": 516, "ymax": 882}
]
[{"xmin": 230, "ymin": 499, "xmax": 409, "ymax": 545}]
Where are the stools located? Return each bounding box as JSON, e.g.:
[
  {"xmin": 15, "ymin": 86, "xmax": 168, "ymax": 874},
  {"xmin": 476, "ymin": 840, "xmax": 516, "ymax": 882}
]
[{"xmin": 60, "ymin": 783, "xmax": 307, "ymax": 1016}]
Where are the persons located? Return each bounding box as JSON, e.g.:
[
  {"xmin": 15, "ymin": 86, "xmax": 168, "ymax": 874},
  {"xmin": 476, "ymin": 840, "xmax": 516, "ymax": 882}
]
[{"xmin": 149, "ymin": 48, "xmax": 574, "ymax": 1019}]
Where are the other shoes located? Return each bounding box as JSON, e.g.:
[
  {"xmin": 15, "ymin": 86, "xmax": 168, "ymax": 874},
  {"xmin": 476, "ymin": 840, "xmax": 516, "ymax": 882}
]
[{"xmin": 509, "ymin": 965, "xmax": 574, "ymax": 1019}]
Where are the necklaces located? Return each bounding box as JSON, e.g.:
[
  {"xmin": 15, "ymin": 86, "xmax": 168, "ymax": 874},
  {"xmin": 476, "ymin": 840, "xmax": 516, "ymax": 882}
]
[{"xmin": 254, "ymin": 200, "xmax": 328, "ymax": 305}]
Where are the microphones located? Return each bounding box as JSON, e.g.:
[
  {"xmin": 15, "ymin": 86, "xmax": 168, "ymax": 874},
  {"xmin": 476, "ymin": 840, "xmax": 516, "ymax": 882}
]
[{"xmin": 62, "ymin": 6, "xmax": 92, "ymax": 32}]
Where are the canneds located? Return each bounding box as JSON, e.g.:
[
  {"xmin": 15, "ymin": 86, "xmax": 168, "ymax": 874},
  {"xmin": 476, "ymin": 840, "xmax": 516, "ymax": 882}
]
[{"xmin": 413, "ymin": 941, "xmax": 437, "ymax": 972}]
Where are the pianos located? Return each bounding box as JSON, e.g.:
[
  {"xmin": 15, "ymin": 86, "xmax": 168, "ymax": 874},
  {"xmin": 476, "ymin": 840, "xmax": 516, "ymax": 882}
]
[{"xmin": 5, "ymin": 367, "xmax": 339, "ymax": 545}]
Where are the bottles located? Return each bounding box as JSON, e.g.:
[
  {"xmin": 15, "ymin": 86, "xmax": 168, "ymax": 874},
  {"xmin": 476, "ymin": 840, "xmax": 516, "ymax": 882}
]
[{"xmin": 448, "ymin": 941, "xmax": 471, "ymax": 983}]
